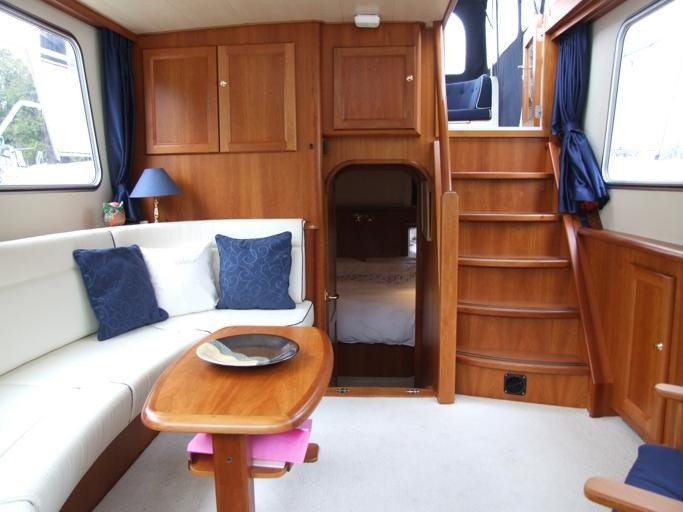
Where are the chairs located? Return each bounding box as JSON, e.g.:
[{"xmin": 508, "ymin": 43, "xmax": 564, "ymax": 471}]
[{"xmin": 584, "ymin": 383, "xmax": 682, "ymax": 512}]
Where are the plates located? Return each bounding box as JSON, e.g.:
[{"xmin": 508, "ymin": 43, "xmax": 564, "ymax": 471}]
[{"xmin": 195, "ymin": 333, "xmax": 300, "ymax": 367}]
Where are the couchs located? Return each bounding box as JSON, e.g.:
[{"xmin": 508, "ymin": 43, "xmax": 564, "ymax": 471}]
[{"xmin": 0, "ymin": 218, "xmax": 314, "ymax": 512}]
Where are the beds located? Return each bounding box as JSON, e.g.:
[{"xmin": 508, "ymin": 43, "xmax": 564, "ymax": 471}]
[{"xmin": 336, "ymin": 256, "xmax": 416, "ymax": 378}]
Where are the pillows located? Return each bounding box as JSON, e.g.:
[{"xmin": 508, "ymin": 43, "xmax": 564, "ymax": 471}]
[{"xmin": 73, "ymin": 230, "xmax": 295, "ymax": 341}]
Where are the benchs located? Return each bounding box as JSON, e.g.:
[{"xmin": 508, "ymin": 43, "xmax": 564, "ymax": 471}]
[{"xmin": 448, "ymin": 74, "xmax": 493, "ymax": 120}]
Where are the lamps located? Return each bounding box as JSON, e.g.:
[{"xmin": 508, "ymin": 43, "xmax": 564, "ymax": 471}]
[{"xmin": 130, "ymin": 167, "xmax": 183, "ymax": 222}]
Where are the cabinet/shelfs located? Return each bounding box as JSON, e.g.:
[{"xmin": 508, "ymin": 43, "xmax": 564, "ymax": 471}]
[
  {"xmin": 142, "ymin": 41, "xmax": 299, "ymax": 156},
  {"xmin": 334, "ymin": 46, "xmax": 417, "ymax": 129}
]
